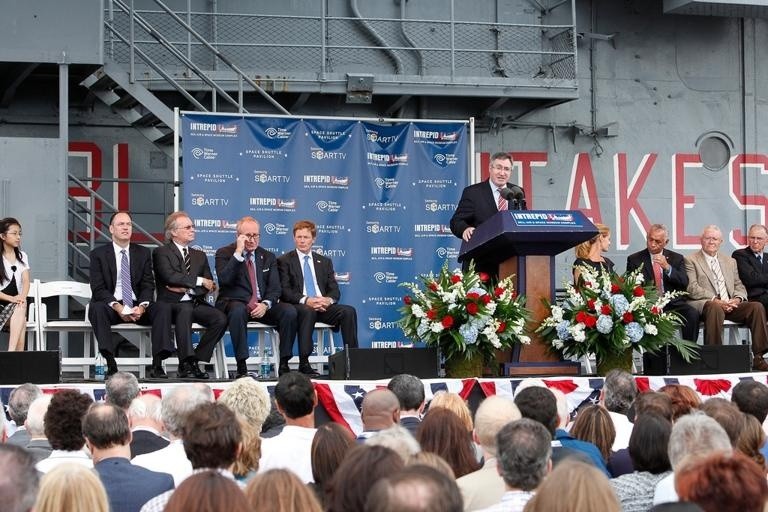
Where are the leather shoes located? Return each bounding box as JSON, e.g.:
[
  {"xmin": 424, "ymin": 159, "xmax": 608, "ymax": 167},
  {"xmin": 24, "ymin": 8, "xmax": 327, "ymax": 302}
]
[
  {"xmin": 177, "ymin": 360, "xmax": 209, "ymax": 378},
  {"xmin": 236, "ymin": 366, "xmax": 247, "ymax": 379},
  {"xmin": 151, "ymin": 368, "xmax": 167, "ymax": 378},
  {"xmin": 299, "ymin": 364, "xmax": 320, "ymax": 376},
  {"xmin": 106, "ymin": 370, "xmax": 117, "ymax": 378},
  {"xmin": 753, "ymin": 358, "xmax": 768, "ymax": 370},
  {"xmin": 279, "ymin": 368, "xmax": 290, "ymax": 377}
]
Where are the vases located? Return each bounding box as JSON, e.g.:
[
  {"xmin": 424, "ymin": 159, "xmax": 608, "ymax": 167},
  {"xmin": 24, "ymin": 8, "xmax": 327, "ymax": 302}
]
[
  {"xmin": 446, "ymin": 352, "xmax": 482, "ymax": 378},
  {"xmin": 596, "ymin": 346, "xmax": 633, "ymax": 377}
]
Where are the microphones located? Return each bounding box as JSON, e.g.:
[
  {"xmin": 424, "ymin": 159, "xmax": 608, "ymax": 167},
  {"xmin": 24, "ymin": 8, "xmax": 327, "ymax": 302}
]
[
  {"xmin": 499, "ymin": 185, "xmax": 519, "ymax": 210},
  {"xmin": 511, "ymin": 187, "xmax": 527, "ymax": 209}
]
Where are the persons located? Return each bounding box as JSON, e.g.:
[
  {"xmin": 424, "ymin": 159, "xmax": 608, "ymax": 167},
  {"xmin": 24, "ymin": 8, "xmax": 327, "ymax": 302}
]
[
  {"xmin": 626, "ymin": 223, "xmax": 701, "ymax": 344},
  {"xmin": 277, "ymin": 220, "xmax": 359, "ymax": 377},
  {"xmin": 0, "ymin": 217, "xmax": 31, "ymax": 351},
  {"xmin": 449, "ymin": 152, "xmax": 528, "ymax": 289},
  {"xmin": 682, "ymin": 225, "xmax": 768, "ymax": 371},
  {"xmin": 151, "ymin": 211, "xmax": 228, "ymax": 379},
  {"xmin": 731, "ymin": 224, "xmax": 768, "ymax": 331},
  {"xmin": 572, "ymin": 221, "xmax": 616, "ymax": 288},
  {"xmin": 214, "ymin": 215, "xmax": 298, "ymax": 378},
  {"xmin": 88, "ymin": 211, "xmax": 175, "ymax": 379},
  {"xmin": 0, "ymin": 366, "xmax": 768, "ymax": 512}
]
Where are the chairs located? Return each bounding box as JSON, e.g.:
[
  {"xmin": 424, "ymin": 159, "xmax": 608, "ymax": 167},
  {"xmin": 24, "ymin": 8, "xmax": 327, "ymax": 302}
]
[{"xmin": 556, "ymin": 288, "xmax": 768, "ymax": 373}]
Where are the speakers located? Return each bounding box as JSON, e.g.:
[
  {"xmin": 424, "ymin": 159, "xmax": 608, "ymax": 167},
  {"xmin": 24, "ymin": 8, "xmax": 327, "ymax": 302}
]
[
  {"xmin": 643, "ymin": 345, "xmax": 754, "ymax": 374},
  {"xmin": 326, "ymin": 347, "xmax": 438, "ymax": 380},
  {"xmin": 0, "ymin": 350, "xmax": 62, "ymax": 384}
]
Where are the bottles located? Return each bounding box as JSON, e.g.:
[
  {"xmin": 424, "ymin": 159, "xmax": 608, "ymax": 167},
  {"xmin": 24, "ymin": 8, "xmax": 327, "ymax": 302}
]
[
  {"xmin": 94, "ymin": 350, "xmax": 106, "ymax": 381},
  {"xmin": 260, "ymin": 347, "xmax": 271, "ymax": 379}
]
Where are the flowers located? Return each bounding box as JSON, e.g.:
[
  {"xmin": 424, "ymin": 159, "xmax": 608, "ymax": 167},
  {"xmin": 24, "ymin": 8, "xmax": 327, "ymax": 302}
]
[
  {"xmin": 534, "ymin": 260, "xmax": 703, "ymax": 368},
  {"xmin": 392, "ymin": 258, "xmax": 541, "ymax": 375}
]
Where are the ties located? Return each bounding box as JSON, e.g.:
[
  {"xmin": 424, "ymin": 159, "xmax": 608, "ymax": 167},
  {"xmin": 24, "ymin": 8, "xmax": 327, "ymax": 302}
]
[
  {"xmin": 497, "ymin": 188, "xmax": 507, "ymax": 211},
  {"xmin": 653, "ymin": 262, "xmax": 662, "ymax": 292},
  {"xmin": 182, "ymin": 248, "xmax": 199, "ymax": 308},
  {"xmin": 121, "ymin": 250, "xmax": 133, "ymax": 309},
  {"xmin": 710, "ymin": 258, "xmax": 730, "ymax": 301},
  {"xmin": 245, "ymin": 253, "xmax": 259, "ymax": 311},
  {"xmin": 303, "ymin": 256, "xmax": 317, "ymax": 299}
]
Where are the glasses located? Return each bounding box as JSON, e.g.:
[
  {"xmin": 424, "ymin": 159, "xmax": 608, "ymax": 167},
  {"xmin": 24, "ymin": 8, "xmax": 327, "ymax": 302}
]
[
  {"xmin": 247, "ymin": 234, "xmax": 260, "ymax": 238},
  {"xmin": 175, "ymin": 224, "xmax": 195, "ymax": 230},
  {"xmin": 6, "ymin": 232, "xmax": 21, "ymax": 235}
]
